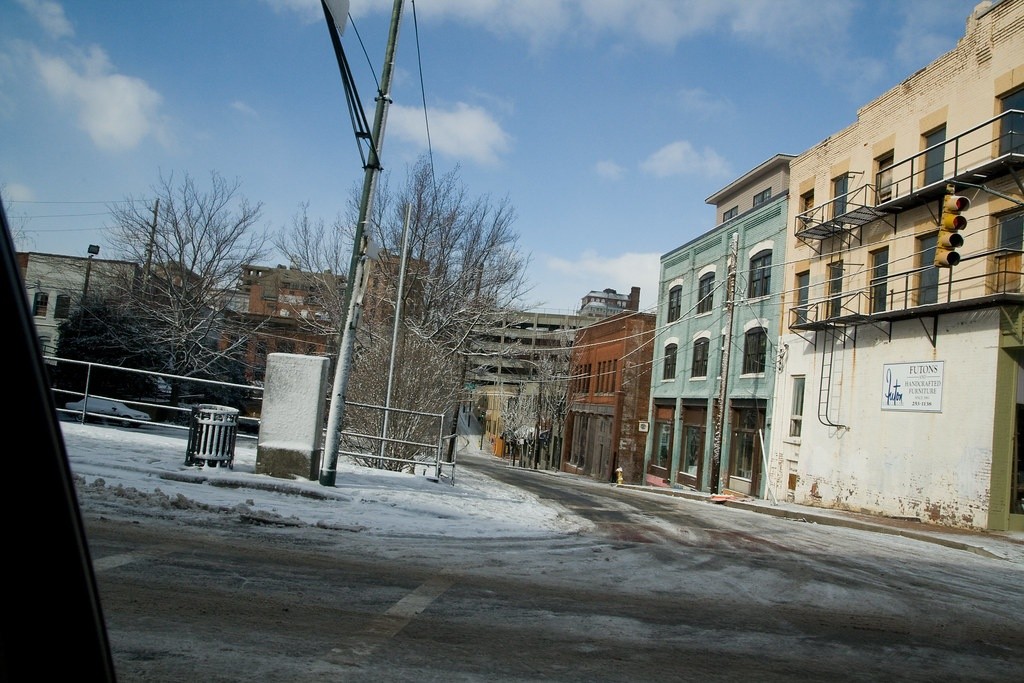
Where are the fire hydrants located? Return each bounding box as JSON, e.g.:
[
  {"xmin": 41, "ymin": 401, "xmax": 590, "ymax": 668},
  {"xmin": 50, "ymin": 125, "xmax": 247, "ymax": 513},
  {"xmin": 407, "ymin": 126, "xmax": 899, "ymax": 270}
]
[{"xmin": 617, "ymin": 467, "xmax": 623, "ymax": 484}]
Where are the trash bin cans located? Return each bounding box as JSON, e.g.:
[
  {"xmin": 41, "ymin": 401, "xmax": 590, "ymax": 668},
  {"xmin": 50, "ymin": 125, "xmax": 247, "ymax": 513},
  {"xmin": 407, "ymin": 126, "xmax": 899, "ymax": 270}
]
[{"xmin": 184, "ymin": 403, "xmax": 242, "ymax": 470}]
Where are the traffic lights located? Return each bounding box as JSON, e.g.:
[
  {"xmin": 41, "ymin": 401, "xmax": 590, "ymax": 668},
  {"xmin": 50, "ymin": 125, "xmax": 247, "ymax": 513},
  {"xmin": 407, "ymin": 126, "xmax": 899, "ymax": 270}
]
[{"xmin": 935, "ymin": 195, "xmax": 971, "ymax": 268}]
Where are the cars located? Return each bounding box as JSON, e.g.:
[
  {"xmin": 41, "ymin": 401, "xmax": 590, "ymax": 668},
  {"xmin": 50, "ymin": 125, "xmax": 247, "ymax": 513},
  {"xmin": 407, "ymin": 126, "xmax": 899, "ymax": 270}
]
[{"xmin": 65, "ymin": 397, "xmax": 152, "ymax": 428}]
